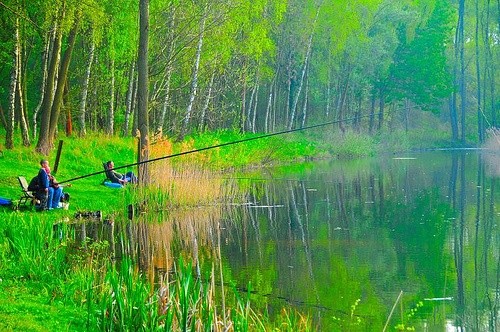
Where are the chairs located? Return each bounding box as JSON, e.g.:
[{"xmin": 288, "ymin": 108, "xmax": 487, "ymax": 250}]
[
  {"xmin": 101, "ymin": 162, "xmax": 128, "ymax": 187},
  {"xmin": 17, "ymin": 176, "xmax": 46, "ymax": 211}
]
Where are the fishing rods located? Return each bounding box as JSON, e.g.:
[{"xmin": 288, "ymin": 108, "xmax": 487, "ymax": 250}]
[
  {"xmin": 49, "ymin": 99, "xmax": 445, "ymax": 190},
  {"xmin": 60, "ymin": 236, "xmax": 379, "ymax": 322}
]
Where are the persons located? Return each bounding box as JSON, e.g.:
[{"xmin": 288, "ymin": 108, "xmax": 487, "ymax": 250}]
[
  {"xmin": 107, "ymin": 161, "xmax": 138, "ymax": 183},
  {"xmin": 45, "ymin": 167, "xmax": 62, "ymax": 209},
  {"xmin": 39, "ymin": 160, "xmax": 49, "ymax": 211}
]
